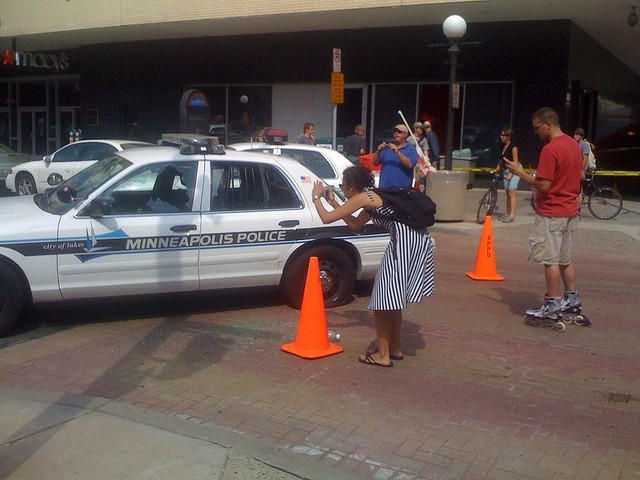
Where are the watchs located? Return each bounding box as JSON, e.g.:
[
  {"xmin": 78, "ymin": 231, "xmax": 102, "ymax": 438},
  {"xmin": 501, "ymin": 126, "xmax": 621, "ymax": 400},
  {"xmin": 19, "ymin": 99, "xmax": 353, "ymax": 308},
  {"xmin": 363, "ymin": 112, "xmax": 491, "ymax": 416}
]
[{"xmin": 312, "ymin": 195, "xmax": 320, "ymax": 202}]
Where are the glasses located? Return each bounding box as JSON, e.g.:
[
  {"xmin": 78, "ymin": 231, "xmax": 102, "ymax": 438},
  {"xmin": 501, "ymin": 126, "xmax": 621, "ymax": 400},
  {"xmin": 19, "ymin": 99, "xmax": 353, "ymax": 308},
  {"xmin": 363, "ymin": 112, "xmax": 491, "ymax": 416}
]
[
  {"xmin": 534, "ymin": 123, "xmax": 546, "ymax": 130},
  {"xmin": 339, "ymin": 184, "xmax": 343, "ymax": 188},
  {"xmin": 393, "ymin": 130, "xmax": 402, "ymax": 133},
  {"xmin": 501, "ymin": 134, "xmax": 507, "ymax": 137}
]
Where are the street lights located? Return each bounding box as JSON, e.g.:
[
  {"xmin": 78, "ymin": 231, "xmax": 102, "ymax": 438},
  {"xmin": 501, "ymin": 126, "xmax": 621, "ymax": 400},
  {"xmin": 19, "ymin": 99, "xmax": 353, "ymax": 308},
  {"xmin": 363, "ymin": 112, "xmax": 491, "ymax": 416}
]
[
  {"xmin": 425, "ymin": 14, "xmax": 469, "ymax": 222},
  {"xmin": 240, "ymin": 95, "xmax": 249, "ymax": 137}
]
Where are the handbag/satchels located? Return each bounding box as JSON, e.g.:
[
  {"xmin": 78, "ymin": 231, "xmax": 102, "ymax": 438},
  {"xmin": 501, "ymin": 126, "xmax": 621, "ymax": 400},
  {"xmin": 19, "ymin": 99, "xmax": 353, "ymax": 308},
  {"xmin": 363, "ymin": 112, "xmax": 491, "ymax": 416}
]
[{"xmin": 364, "ymin": 187, "xmax": 436, "ymax": 229}]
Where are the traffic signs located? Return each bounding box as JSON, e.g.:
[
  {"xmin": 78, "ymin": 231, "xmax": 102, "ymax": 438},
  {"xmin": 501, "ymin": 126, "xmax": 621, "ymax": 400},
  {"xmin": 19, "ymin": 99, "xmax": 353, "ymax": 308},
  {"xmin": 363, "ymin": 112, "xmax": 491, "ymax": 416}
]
[{"xmin": 452, "ymin": 83, "xmax": 461, "ymax": 110}]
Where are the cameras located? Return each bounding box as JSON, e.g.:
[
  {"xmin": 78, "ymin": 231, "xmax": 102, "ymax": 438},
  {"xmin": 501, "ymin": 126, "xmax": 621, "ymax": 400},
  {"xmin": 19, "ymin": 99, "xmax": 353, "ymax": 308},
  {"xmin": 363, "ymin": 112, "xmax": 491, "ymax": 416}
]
[
  {"xmin": 386, "ymin": 143, "xmax": 390, "ymax": 148},
  {"xmin": 323, "ymin": 187, "xmax": 329, "ymax": 198}
]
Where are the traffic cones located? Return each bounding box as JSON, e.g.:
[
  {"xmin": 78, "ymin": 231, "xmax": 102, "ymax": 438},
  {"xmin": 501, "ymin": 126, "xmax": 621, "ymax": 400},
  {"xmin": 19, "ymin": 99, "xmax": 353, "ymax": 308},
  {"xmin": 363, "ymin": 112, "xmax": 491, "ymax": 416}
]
[
  {"xmin": 464, "ymin": 214, "xmax": 505, "ymax": 282},
  {"xmin": 280, "ymin": 255, "xmax": 345, "ymax": 358}
]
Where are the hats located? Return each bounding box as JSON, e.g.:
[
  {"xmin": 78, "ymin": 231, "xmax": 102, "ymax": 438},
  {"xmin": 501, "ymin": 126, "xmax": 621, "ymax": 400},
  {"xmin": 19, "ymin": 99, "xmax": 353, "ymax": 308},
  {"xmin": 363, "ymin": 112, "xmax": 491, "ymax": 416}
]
[
  {"xmin": 575, "ymin": 129, "xmax": 585, "ymax": 135},
  {"xmin": 394, "ymin": 125, "xmax": 407, "ymax": 131},
  {"xmin": 413, "ymin": 122, "xmax": 424, "ymax": 128}
]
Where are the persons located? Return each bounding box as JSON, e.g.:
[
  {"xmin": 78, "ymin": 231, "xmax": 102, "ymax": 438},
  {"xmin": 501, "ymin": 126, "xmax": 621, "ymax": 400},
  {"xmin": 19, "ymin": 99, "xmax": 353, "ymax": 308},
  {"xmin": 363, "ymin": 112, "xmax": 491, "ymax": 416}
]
[
  {"xmin": 312, "ymin": 166, "xmax": 435, "ymax": 366},
  {"xmin": 503, "ymin": 107, "xmax": 592, "ymax": 332},
  {"xmin": 575, "ymin": 128, "xmax": 597, "ymax": 216},
  {"xmin": 372, "ymin": 125, "xmax": 419, "ymax": 192},
  {"xmin": 423, "ymin": 122, "xmax": 441, "ymax": 169},
  {"xmin": 343, "ymin": 125, "xmax": 367, "ymax": 159},
  {"xmin": 297, "ymin": 124, "xmax": 316, "ymax": 144},
  {"xmin": 490, "ymin": 128, "xmax": 523, "ymax": 223},
  {"xmin": 407, "ymin": 122, "xmax": 428, "ymax": 156}
]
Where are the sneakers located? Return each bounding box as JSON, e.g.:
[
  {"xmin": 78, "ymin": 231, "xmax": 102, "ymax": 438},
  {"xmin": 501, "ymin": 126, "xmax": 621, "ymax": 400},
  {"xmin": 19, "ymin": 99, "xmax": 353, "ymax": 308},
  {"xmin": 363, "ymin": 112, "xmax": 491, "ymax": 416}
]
[
  {"xmin": 498, "ymin": 214, "xmax": 508, "ymax": 221},
  {"xmin": 502, "ymin": 216, "xmax": 513, "ymax": 223}
]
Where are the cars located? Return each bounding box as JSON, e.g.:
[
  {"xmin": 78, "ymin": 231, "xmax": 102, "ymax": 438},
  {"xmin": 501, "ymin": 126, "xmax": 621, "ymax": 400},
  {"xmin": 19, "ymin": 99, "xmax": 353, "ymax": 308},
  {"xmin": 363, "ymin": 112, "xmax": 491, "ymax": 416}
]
[
  {"xmin": 4, "ymin": 138, "xmax": 160, "ymax": 197},
  {"xmin": 0, "ymin": 132, "xmax": 434, "ymax": 337},
  {"xmin": 225, "ymin": 127, "xmax": 382, "ymax": 201}
]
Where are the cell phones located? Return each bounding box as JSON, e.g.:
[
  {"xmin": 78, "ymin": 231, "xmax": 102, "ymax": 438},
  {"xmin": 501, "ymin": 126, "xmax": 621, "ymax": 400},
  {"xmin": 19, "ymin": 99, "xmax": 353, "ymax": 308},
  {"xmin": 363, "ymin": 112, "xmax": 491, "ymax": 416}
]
[{"xmin": 501, "ymin": 152, "xmax": 510, "ymax": 162}]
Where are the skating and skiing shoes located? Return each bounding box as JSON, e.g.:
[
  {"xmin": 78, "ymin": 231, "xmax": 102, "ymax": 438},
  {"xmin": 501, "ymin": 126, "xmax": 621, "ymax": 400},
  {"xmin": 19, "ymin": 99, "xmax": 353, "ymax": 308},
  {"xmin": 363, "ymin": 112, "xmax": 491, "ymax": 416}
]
[
  {"xmin": 561, "ymin": 292, "xmax": 591, "ymax": 326},
  {"xmin": 524, "ymin": 293, "xmax": 565, "ymax": 332}
]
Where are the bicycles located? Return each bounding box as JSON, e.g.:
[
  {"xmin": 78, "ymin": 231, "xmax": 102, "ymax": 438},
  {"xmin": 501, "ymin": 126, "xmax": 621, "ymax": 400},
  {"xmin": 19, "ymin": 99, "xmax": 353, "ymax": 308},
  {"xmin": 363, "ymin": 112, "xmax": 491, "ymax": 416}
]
[
  {"xmin": 531, "ymin": 168, "xmax": 623, "ymax": 219},
  {"xmin": 475, "ymin": 170, "xmax": 507, "ymax": 225}
]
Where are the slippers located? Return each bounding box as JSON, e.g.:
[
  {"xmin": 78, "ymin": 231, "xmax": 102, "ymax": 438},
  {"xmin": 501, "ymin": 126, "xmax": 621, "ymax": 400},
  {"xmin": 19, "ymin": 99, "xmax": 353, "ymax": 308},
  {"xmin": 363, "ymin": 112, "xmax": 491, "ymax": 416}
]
[
  {"xmin": 359, "ymin": 354, "xmax": 393, "ymax": 367},
  {"xmin": 368, "ymin": 348, "xmax": 403, "ymax": 360}
]
